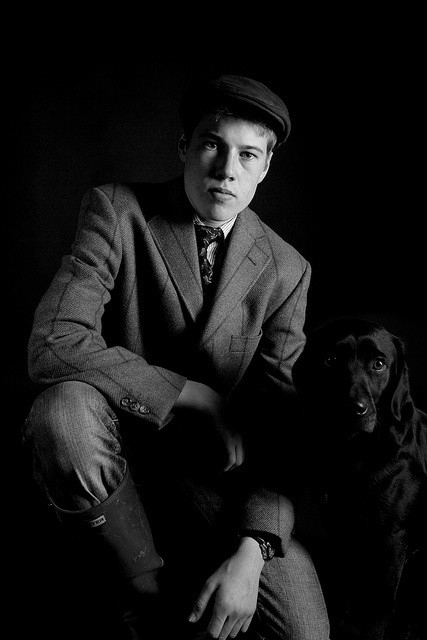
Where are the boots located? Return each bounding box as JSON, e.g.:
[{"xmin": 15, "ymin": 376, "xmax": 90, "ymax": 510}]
[{"xmin": 45, "ymin": 457, "xmax": 164, "ymax": 640}]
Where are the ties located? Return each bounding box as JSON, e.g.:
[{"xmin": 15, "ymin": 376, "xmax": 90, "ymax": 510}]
[{"xmin": 194, "ymin": 224, "xmax": 224, "ymax": 286}]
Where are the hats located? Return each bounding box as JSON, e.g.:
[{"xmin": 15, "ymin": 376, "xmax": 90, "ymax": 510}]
[{"xmin": 180, "ymin": 75, "xmax": 292, "ymax": 152}]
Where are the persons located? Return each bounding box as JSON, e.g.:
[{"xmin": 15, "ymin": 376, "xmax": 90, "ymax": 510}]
[{"xmin": 15, "ymin": 73, "xmax": 333, "ymax": 639}]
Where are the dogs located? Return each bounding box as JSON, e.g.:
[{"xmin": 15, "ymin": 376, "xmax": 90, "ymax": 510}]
[{"xmin": 305, "ymin": 327, "xmax": 426, "ymax": 640}]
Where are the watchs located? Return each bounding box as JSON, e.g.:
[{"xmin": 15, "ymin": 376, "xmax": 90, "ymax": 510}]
[{"xmin": 250, "ymin": 532, "xmax": 278, "ymax": 561}]
